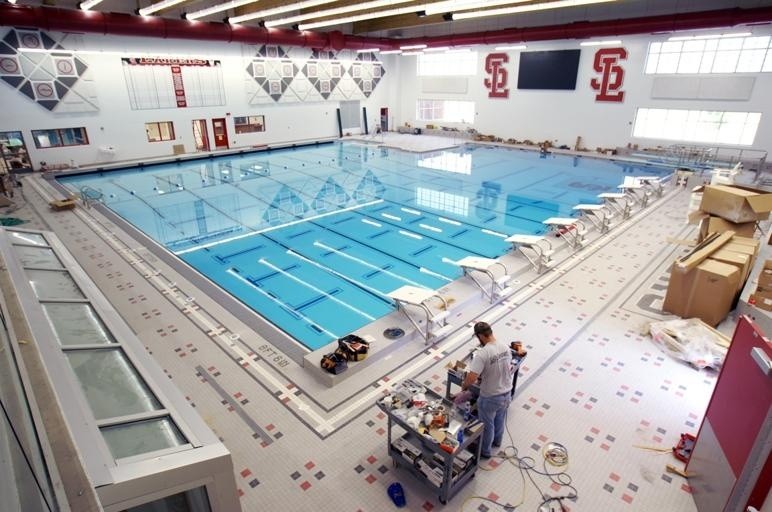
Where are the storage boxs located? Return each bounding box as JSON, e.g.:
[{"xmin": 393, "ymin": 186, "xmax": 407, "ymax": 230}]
[{"xmin": 662, "ymin": 183, "xmax": 772, "ymax": 328}]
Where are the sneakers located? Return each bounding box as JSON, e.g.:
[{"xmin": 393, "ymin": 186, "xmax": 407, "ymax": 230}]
[
  {"xmin": 468, "ymin": 443, "xmax": 492, "ymax": 459},
  {"xmin": 476, "ymin": 435, "xmax": 502, "ymax": 447}
]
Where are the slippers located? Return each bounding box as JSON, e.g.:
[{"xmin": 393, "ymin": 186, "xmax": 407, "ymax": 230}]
[{"xmin": 388, "ymin": 482, "xmax": 406, "ymax": 507}]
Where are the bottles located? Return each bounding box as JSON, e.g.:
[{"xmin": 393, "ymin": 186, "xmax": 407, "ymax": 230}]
[
  {"xmin": 458, "ymin": 430, "xmax": 463, "ymax": 443},
  {"xmin": 464, "ymin": 402, "xmax": 470, "ymax": 421},
  {"xmin": 443, "ymin": 411, "xmax": 449, "ymax": 428}
]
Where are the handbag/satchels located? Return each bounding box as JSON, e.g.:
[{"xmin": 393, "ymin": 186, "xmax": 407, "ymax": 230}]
[
  {"xmin": 321, "ymin": 353, "xmax": 348, "ymax": 375},
  {"xmin": 335, "ymin": 334, "xmax": 369, "ymax": 362}
]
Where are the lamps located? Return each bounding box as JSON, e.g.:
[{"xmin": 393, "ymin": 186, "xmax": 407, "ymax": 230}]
[{"xmin": 78, "ymin": 0, "xmax": 621, "ymax": 56}]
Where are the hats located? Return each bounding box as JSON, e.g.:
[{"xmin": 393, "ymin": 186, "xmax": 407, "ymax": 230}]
[{"xmin": 472, "ymin": 322, "xmax": 491, "ymax": 337}]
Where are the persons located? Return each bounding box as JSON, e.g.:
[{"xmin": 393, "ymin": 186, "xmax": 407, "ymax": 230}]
[{"xmin": 462, "ymin": 322, "xmax": 513, "ymax": 458}]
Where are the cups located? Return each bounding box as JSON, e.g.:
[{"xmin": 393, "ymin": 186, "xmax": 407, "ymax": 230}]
[{"xmin": 384, "ymin": 397, "xmax": 392, "ymax": 411}]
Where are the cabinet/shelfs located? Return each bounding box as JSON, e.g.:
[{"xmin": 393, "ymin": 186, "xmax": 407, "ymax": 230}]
[
  {"xmin": 376, "ymin": 380, "xmax": 485, "ymax": 505},
  {"xmin": 446, "ymin": 345, "xmax": 525, "ymax": 416}
]
